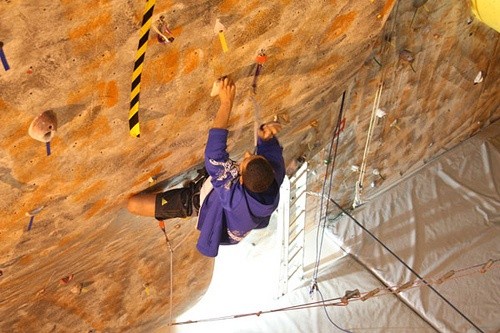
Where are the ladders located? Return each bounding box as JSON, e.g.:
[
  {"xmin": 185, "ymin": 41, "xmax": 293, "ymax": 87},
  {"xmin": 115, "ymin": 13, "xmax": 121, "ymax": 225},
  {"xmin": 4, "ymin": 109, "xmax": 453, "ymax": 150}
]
[{"xmin": 277, "ymin": 158, "xmax": 309, "ymax": 297}]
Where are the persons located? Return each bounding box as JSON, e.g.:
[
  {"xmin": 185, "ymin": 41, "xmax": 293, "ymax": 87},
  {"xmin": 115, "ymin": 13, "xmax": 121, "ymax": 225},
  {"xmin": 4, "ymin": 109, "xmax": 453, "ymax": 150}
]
[{"xmin": 125, "ymin": 76, "xmax": 286, "ymax": 262}]
